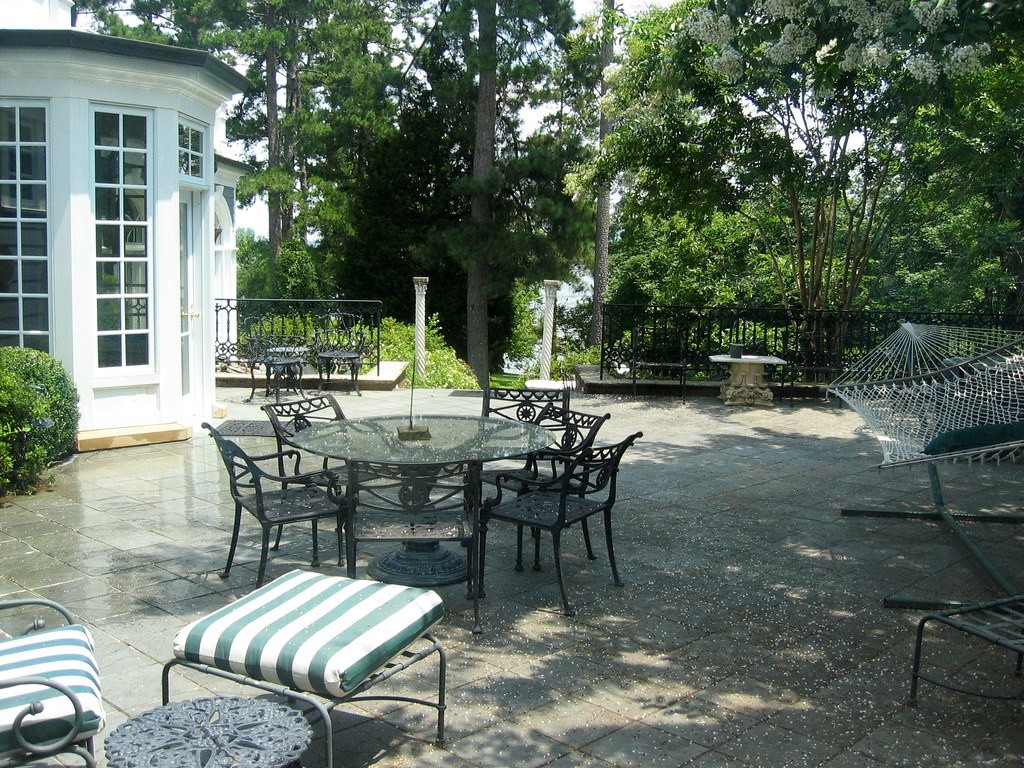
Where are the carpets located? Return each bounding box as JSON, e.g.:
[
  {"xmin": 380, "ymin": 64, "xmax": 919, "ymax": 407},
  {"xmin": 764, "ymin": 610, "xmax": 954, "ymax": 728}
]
[{"xmin": 209, "ymin": 419, "xmax": 327, "ymax": 437}]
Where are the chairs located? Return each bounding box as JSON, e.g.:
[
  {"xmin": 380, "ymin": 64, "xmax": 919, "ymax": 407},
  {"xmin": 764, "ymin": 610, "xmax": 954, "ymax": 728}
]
[
  {"xmin": 463, "ymin": 402, "xmax": 611, "ymax": 572},
  {"xmin": 478, "ymin": 431, "xmax": 644, "ymax": 614},
  {"xmin": 260, "ymin": 394, "xmax": 400, "ymax": 566},
  {"xmin": 201, "ymin": 422, "xmax": 346, "ymax": 590},
  {"xmin": 311, "ymin": 309, "xmax": 374, "ymax": 396},
  {"xmin": 239, "ymin": 313, "xmax": 308, "ymax": 405},
  {"xmin": 632, "ymin": 326, "xmax": 688, "ymax": 405},
  {"xmin": 0, "ymin": 598, "xmax": 108, "ymax": 768},
  {"xmin": 461, "ymin": 387, "xmax": 571, "ymax": 507}
]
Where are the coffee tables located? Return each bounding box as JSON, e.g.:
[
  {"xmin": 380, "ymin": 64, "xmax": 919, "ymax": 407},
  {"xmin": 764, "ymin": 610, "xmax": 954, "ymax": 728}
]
[{"xmin": 103, "ymin": 697, "xmax": 313, "ymax": 768}]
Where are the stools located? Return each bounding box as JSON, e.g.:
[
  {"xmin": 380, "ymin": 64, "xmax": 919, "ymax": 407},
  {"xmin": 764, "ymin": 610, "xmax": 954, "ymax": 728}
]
[{"xmin": 162, "ymin": 569, "xmax": 447, "ymax": 768}]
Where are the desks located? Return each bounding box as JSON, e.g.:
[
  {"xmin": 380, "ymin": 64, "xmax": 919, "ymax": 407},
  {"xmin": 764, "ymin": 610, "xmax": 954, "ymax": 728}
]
[
  {"xmin": 293, "ymin": 415, "xmax": 557, "ymax": 634},
  {"xmin": 708, "ymin": 355, "xmax": 787, "ymax": 407},
  {"xmin": 265, "ymin": 346, "xmax": 312, "ymax": 397}
]
